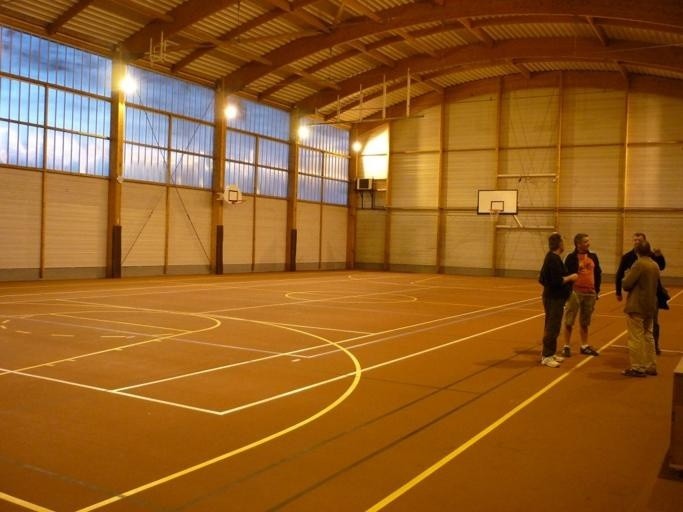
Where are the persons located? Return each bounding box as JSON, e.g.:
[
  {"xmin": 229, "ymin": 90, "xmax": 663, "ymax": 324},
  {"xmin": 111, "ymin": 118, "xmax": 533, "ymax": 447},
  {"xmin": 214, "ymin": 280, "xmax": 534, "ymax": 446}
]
[
  {"xmin": 537, "ymin": 233, "xmax": 579, "ymax": 368},
  {"xmin": 562, "ymin": 233, "xmax": 602, "ymax": 357},
  {"xmin": 622, "ymin": 240, "xmax": 661, "ymax": 377},
  {"xmin": 615, "ymin": 232, "xmax": 670, "ymax": 355}
]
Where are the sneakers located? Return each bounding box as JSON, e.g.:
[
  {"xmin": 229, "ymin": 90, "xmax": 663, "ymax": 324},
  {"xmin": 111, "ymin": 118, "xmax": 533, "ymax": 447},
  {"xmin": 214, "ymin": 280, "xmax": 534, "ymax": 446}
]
[
  {"xmin": 540, "ymin": 354, "xmax": 564, "ymax": 367},
  {"xmin": 622, "ymin": 368, "xmax": 656, "ymax": 377},
  {"xmin": 580, "ymin": 346, "xmax": 599, "ymax": 356},
  {"xmin": 563, "ymin": 347, "xmax": 570, "ymax": 356}
]
